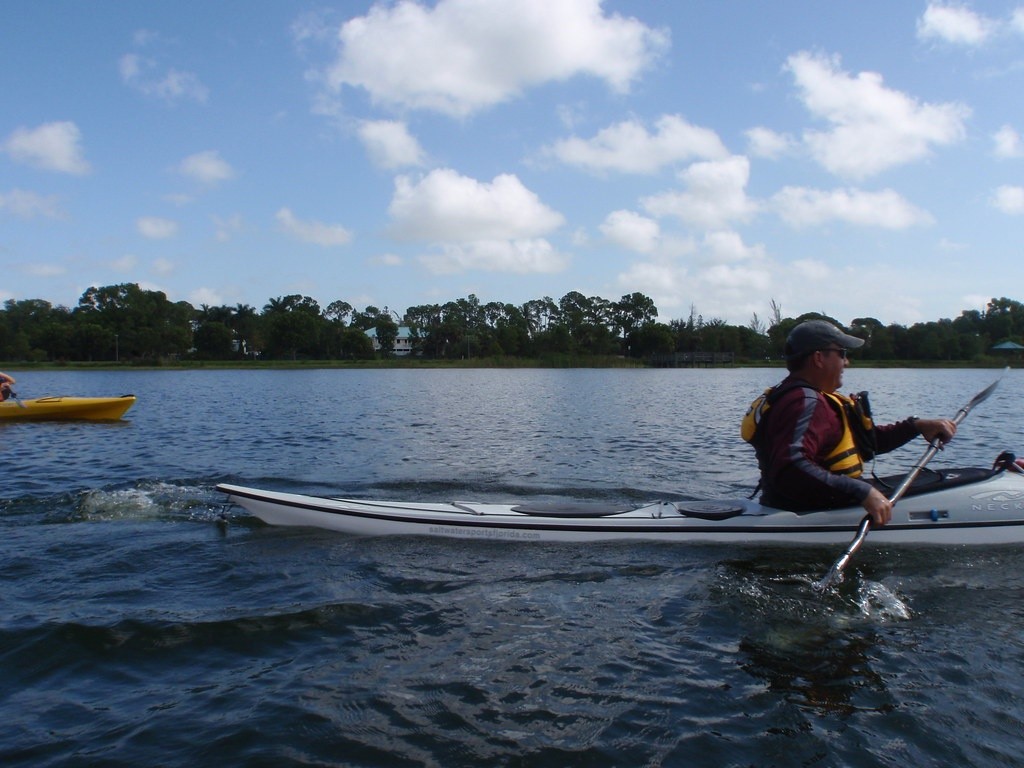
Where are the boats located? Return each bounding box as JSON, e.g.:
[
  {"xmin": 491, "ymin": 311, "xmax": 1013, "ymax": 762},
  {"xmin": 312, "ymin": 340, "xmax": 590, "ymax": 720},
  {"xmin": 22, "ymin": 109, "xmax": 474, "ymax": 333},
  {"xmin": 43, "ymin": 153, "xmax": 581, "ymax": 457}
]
[
  {"xmin": 216, "ymin": 454, "xmax": 1024, "ymax": 545},
  {"xmin": 0, "ymin": 394, "xmax": 136, "ymax": 420}
]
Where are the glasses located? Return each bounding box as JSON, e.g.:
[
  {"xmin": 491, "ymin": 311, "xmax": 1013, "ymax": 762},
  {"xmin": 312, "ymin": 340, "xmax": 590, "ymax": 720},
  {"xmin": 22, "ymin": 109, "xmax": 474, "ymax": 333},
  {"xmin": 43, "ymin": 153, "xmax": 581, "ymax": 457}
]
[{"xmin": 810, "ymin": 349, "xmax": 847, "ymax": 360}]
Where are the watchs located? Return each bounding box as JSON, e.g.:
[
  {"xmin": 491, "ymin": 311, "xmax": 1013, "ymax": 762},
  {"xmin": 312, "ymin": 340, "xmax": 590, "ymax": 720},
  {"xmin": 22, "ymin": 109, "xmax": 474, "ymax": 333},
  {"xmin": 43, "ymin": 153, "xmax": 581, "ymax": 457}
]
[{"xmin": 907, "ymin": 415, "xmax": 920, "ymax": 437}]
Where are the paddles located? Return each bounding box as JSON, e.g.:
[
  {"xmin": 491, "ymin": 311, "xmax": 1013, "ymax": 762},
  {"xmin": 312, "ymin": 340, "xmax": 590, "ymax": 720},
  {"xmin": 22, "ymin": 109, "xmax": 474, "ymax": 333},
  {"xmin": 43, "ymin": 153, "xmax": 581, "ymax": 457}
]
[
  {"xmin": 812, "ymin": 364, "xmax": 1014, "ymax": 588},
  {"xmin": 6, "ymin": 387, "xmax": 27, "ymax": 409}
]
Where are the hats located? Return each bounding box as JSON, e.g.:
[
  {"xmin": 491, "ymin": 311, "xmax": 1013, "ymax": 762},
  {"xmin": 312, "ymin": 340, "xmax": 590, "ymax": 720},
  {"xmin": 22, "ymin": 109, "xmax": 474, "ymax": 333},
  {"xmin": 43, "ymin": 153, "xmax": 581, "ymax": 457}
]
[{"xmin": 784, "ymin": 320, "xmax": 865, "ymax": 356}]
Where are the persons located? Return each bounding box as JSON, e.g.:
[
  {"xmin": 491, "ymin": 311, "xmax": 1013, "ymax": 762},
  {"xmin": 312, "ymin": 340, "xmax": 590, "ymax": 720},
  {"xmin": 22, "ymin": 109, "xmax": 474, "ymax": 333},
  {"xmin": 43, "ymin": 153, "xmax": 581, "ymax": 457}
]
[
  {"xmin": 0, "ymin": 372, "xmax": 16, "ymax": 402},
  {"xmin": 741, "ymin": 320, "xmax": 957, "ymax": 529}
]
